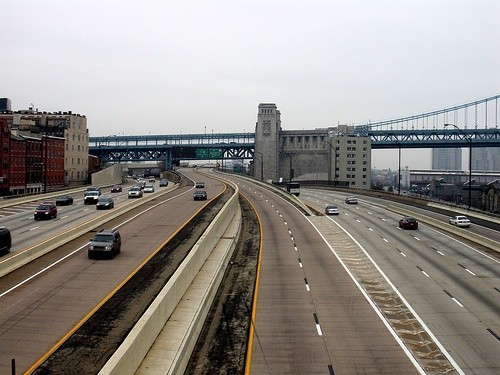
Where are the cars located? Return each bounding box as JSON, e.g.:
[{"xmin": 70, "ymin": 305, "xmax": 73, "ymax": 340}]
[
  {"xmin": 193, "ymin": 190, "xmax": 207, "ymax": 201},
  {"xmin": 143, "ymin": 185, "xmax": 154, "ymax": 193},
  {"xmin": 449, "ymin": 215, "xmax": 471, "ymax": 228},
  {"xmin": 324, "ymin": 205, "xmax": 339, "ymax": 215},
  {"xmin": 110, "ymin": 185, "xmax": 122, "ymax": 193},
  {"xmin": 56, "ymin": 195, "xmax": 74, "ymax": 206},
  {"xmin": 196, "ymin": 181, "xmax": 205, "ymax": 188},
  {"xmin": 345, "ymin": 196, "xmax": 358, "ymax": 204},
  {"xmin": 126, "ymin": 173, "xmax": 169, "ymax": 190},
  {"xmin": 96, "ymin": 197, "xmax": 114, "ymax": 210}
]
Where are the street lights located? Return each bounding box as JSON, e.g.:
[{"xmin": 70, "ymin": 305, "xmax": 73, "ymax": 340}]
[{"xmin": 444, "ymin": 123, "xmax": 472, "ymax": 211}]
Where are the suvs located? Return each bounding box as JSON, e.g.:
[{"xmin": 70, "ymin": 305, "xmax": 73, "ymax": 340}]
[
  {"xmin": 128, "ymin": 186, "xmax": 143, "ymax": 198},
  {"xmin": 0, "ymin": 225, "xmax": 12, "ymax": 256},
  {"xmin": 88, "ymin": 228, "xmax": 122, "ymax": 260},
  {"xmin": 34, "ymin": 201, "xmax": 58, "ymax": 220},
  {"xmin": 399, "ymin": 216, "xmax": 419, "ymax": 230},
  {"xmin": 83, "ymin": 190, "xmax": 100, "ymax": 205},
  {"xmin": 87, "ymin": 187, "xmax": 101, "ymax": 195},
  {"xmin": 285, "ymin": 183, "xmax": 300, "ymax": 196}
]
